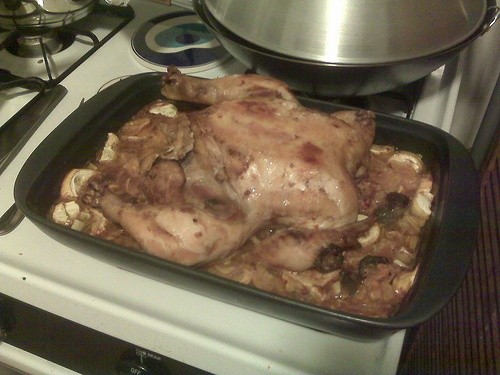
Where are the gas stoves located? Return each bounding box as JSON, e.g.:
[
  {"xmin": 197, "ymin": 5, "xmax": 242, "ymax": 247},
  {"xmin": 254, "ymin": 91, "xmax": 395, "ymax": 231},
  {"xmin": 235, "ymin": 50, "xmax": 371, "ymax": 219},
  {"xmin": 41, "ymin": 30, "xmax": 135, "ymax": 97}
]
[{"xmin": 0, "ymin": 0, "xmax": 471, "ymax": 375}]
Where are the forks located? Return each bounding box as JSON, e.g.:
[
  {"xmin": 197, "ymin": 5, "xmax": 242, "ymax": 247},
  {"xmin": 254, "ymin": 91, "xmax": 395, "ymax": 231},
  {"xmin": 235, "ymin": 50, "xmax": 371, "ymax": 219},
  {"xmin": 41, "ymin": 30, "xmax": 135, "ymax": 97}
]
[{"xmin": 0, "ymin": 97, "xmax": 86, "ymax": 237}]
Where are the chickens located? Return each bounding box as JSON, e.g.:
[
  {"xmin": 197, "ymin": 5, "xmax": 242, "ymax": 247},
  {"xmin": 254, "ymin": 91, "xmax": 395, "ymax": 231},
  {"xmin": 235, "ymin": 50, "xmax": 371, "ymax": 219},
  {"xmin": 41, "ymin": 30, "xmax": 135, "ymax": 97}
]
[{"xmin": 83, "ymin": 64, "xmax": 420, "ymax": 273}]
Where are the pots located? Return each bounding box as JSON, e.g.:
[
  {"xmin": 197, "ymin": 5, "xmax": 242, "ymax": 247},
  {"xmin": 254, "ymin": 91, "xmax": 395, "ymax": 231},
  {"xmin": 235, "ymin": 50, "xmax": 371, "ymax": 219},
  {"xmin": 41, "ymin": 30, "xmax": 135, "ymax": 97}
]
[{"xmin": 155, "ymin": 0, "xmax": 500, "ymax": 101}]
